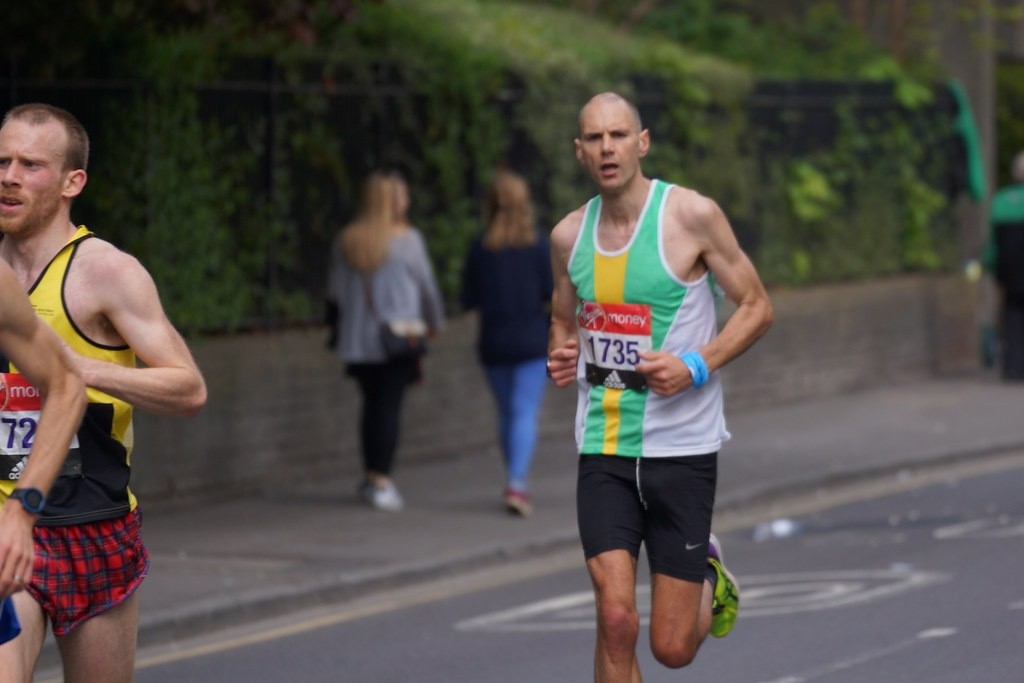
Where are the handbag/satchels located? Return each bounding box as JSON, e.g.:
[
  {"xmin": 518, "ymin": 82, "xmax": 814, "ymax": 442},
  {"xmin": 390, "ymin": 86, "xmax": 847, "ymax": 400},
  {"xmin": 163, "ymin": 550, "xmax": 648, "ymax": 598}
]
[{"xmin": 381, "ymin": 319, "xmax": 428, "ymax": 357}]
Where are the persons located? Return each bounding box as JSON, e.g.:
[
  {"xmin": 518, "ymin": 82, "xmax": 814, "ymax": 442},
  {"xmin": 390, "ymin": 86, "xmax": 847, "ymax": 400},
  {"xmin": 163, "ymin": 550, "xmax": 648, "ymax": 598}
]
[
  {"xmin": 547, "ymin": 92, "xmax": 775, "ymax": 683},
  {"xmin": 459, "ymin": 170, "xmax": 559, "ymax": 517},
  {"xmin": 323, "ymin": 168, "xmax": 447, "ymax": 515},
  {"xmin": 0, "ymin": 102, "xmax": 208, "ymax": 683}
]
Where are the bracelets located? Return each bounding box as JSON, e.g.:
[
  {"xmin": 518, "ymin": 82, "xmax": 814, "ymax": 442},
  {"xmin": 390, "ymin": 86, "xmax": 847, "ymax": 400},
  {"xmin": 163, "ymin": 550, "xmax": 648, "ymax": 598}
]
[
  {"xmin": 680, "ymin": 351, "xmax": 709, "ymax": 388},
  {"xmin": 546, "ymin": 360, "xmax": 551, "ymax": 377}
]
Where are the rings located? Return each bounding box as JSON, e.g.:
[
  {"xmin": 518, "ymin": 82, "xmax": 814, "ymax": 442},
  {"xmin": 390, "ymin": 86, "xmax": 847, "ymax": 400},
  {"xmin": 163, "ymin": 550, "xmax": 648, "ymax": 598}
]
[{"xmin": 14, "ymin": 576, "xmax": 23, "ymax": 581}]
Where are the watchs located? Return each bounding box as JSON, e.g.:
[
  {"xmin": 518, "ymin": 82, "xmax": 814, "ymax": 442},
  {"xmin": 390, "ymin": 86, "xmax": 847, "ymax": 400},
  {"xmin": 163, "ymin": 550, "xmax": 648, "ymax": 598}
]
[{"xmin": 9, "ymin": 487, "xmax": 47, "ymax": 514}]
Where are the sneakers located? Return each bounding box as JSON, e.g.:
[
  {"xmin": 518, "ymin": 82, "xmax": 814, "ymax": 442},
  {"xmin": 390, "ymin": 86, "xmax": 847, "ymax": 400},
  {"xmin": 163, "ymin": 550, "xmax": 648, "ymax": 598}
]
[{"xmin": 706, "ymin": 533, "xmax": 739, "ymax": 639}]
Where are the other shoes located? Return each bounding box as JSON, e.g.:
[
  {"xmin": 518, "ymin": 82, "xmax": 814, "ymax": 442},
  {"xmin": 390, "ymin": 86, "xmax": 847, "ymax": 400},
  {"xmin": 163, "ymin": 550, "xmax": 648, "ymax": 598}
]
[
  {"xmin": 362, "ymin": 480, "xmax": 404, "ymax": 511},
  {"xmin": 503, "ymin": 489, "xmax": 531, "ymax": 516}
]
